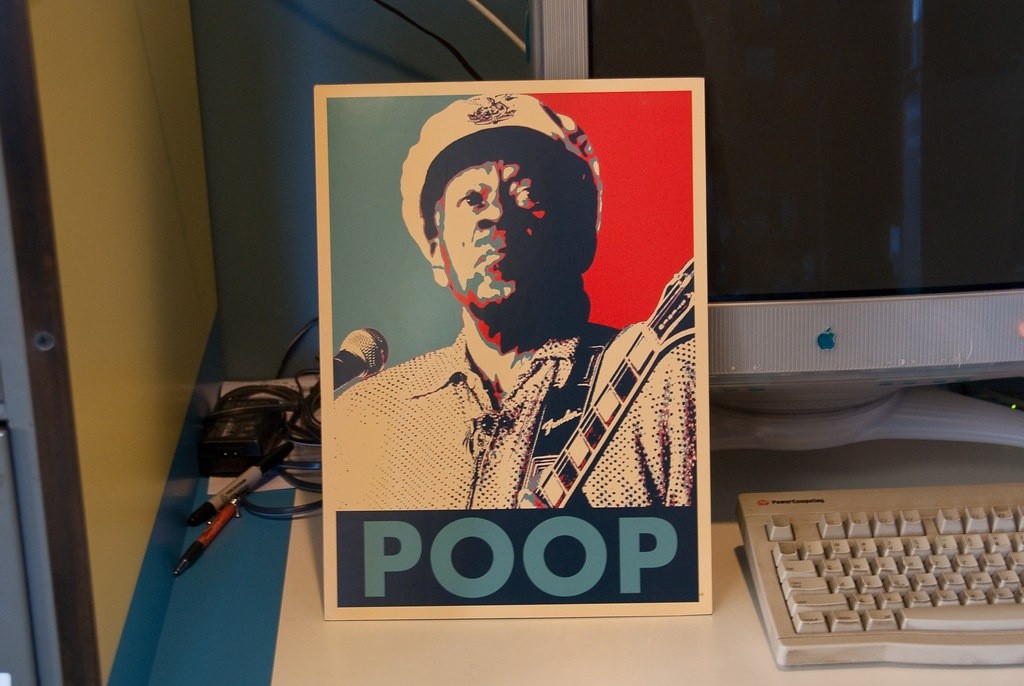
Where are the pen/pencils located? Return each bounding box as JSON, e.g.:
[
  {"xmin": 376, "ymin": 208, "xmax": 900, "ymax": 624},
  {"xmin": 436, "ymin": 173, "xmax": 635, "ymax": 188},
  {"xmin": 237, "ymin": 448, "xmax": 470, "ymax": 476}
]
[{"xmin": 174, "ymin": 498, "xmax": 239, "ymax": 577}]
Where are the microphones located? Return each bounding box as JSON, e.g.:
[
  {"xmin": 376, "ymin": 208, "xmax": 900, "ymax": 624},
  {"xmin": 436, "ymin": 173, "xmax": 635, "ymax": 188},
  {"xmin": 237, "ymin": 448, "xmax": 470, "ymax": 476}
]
[{"xmin": 332, "ymin": 327, "xmax": 389, "ymax": 391}]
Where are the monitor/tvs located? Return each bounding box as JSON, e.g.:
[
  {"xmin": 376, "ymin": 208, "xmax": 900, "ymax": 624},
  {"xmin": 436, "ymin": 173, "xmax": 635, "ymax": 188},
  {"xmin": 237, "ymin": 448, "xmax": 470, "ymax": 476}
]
[{"xmin": 536, "ymin": 0, "xmax": 1023, "ymax": 450}]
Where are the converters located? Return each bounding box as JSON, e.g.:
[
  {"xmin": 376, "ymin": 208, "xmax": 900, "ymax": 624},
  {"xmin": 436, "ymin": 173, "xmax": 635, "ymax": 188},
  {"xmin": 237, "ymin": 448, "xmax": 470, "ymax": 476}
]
[{"xmin": 196, "ymin": 398, "xmax": 283, "ymax": 478}]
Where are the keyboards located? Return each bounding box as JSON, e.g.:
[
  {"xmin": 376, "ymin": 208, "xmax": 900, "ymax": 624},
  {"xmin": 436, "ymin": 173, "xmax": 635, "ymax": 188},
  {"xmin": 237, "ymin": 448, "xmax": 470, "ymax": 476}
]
[{"xmin": 736, "ymin": 481, "xmax": 1024, "ymax": 667}]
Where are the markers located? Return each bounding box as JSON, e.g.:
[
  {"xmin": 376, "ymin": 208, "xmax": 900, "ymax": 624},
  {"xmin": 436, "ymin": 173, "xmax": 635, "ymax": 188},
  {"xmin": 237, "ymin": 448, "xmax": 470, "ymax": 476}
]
[{"xmin": 187, "ymin": 442, "xmax": 295, "ymax": 528}]
